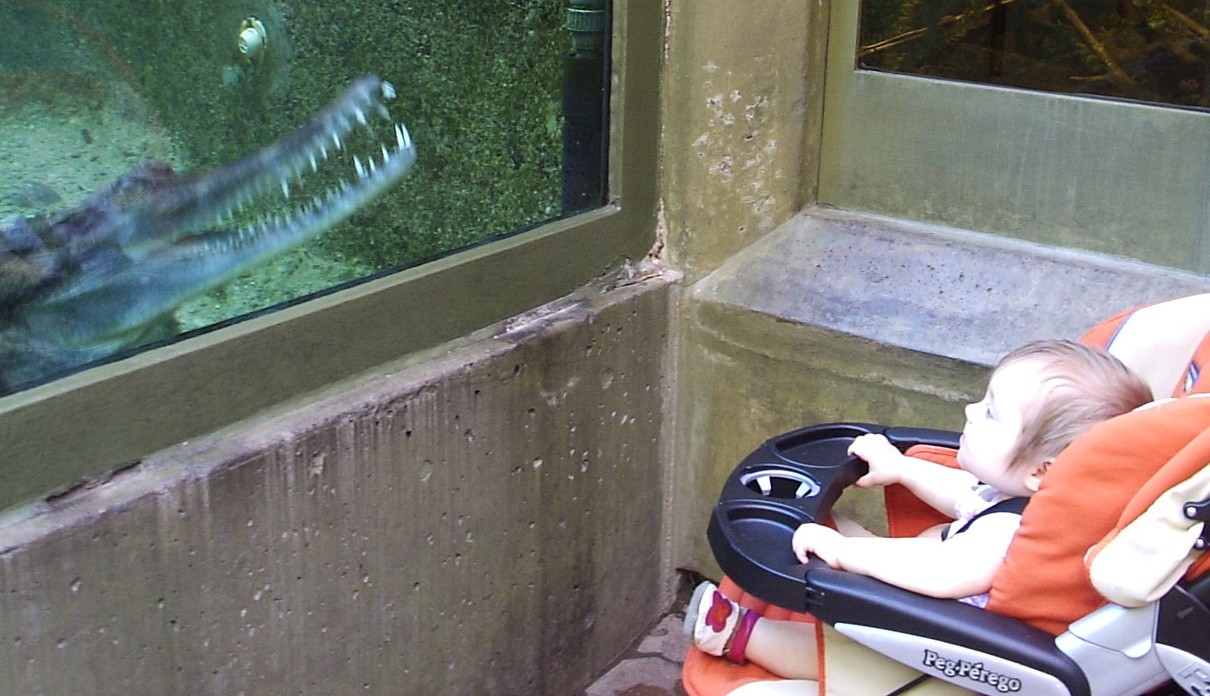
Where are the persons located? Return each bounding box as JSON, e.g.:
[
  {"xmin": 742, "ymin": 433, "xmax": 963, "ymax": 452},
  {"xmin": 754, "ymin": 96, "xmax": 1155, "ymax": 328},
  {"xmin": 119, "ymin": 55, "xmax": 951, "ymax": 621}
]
[{"xmin": 682, "ymin": 337, "xmax": 1153, "ymax": 680}]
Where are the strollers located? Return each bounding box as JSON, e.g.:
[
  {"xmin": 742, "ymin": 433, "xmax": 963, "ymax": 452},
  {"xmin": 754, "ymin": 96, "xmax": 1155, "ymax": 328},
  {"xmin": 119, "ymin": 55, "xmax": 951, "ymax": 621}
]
[{"xmin": 681, "ymin": 287, "xmax": 1210, "ymax": 696}]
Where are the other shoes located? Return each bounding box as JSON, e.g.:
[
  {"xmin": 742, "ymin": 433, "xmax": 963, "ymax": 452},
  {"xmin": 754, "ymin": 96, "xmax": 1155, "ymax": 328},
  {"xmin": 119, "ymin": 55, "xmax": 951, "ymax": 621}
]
[{"xmin": 684, "ymin": 581, "xmax": 739, "ymax": 656}]
